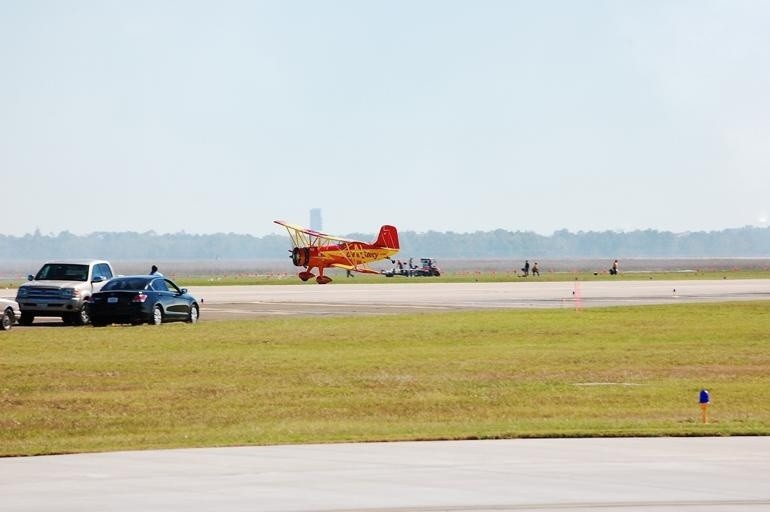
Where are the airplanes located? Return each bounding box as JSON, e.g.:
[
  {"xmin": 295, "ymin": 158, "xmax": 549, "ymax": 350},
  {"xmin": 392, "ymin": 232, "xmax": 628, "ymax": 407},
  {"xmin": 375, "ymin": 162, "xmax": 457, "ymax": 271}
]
[{"xmin": 274, "ymin": 220, "xmax": 408, "ymax": 285}]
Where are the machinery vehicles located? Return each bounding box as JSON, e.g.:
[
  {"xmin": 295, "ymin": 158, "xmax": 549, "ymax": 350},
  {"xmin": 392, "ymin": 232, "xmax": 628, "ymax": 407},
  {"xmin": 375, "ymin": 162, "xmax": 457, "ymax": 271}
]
[{"xmin": 413, "ymin": 258, "xmax": 441, "ymax": 277}]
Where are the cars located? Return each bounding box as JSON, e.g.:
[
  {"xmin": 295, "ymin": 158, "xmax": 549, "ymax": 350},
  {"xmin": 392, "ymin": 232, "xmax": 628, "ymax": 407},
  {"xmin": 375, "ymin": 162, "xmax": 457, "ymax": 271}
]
[
  {"xmin": 87, "ymin": 276, "xmax": 199, "ymax": 326},
  {"xmin": 0, "ymin": 299, "xmax": 21, "ymax": 330}
]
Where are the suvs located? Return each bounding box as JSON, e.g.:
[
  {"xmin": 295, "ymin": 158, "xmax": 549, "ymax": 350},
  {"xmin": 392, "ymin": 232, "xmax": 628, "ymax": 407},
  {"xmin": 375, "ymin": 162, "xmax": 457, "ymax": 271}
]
[{"xmin": 16, "ymin": 261, "xmax": 115, "ymax": 325}]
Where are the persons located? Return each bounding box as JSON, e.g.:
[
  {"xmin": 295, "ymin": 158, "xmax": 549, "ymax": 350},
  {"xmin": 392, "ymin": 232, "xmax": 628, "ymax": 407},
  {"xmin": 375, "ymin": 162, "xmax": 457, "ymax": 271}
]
[
  {"xmin": 525, "ymin": 260, "xmax": 529, "ymax": 275},
  {"xmin": 532, "ymin": 263, "xmax": 540, "ymax": 276},
  {"xmin": 613, "ymin": 260, "xmax": 619, "ymax": 274},
  {"xmin": 149, "ymin": 264, "xmax": 163, "ymax": 277}
]
